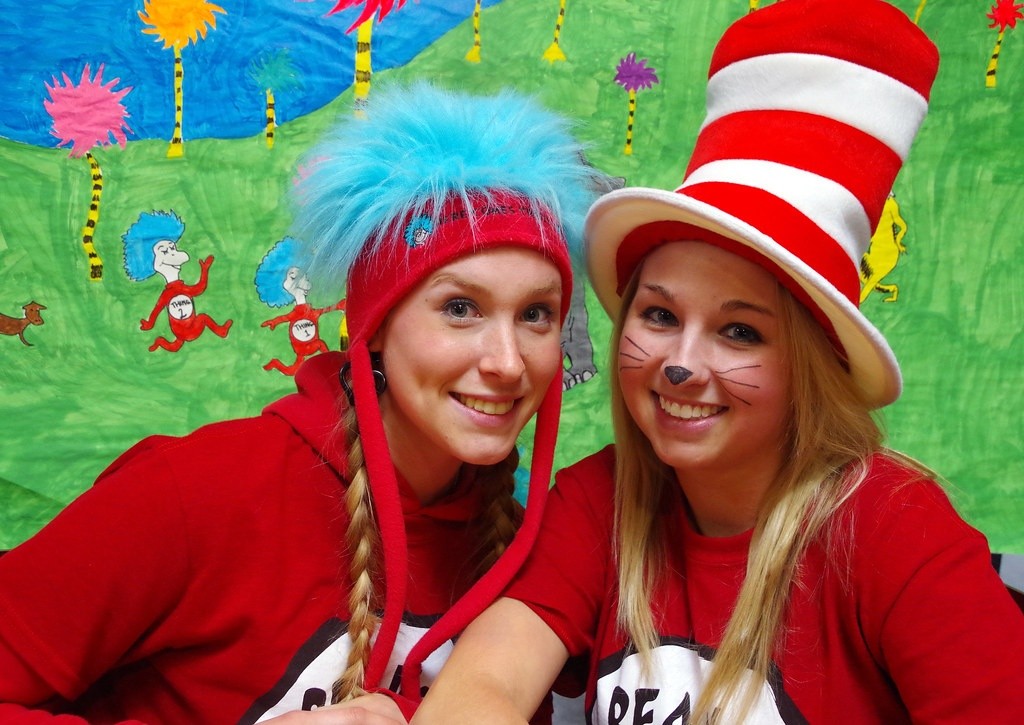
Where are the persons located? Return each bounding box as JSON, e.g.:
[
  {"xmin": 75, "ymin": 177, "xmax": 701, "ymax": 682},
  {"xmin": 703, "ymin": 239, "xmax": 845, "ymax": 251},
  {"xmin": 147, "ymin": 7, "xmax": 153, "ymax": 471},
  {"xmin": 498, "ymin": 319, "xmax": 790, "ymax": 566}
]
[
  {"xmin": 1, "ymin": 89, "xmax": 605, "ymax": 725},
  {"xmin": 405, "ymin": 179, "xmax": 1024, "ymax": 724}
]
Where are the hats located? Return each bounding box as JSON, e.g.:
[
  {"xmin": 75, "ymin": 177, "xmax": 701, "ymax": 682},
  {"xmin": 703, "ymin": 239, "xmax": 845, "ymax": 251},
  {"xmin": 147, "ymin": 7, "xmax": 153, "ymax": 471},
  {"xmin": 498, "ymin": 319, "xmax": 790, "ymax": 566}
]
[
  {"xmin": 289, "ymin": 78, "xmax": 615, "ymax": 346},
  {"xmin": 584, "ymin": 0, "xmax": 937, "ymax": 411}
]
